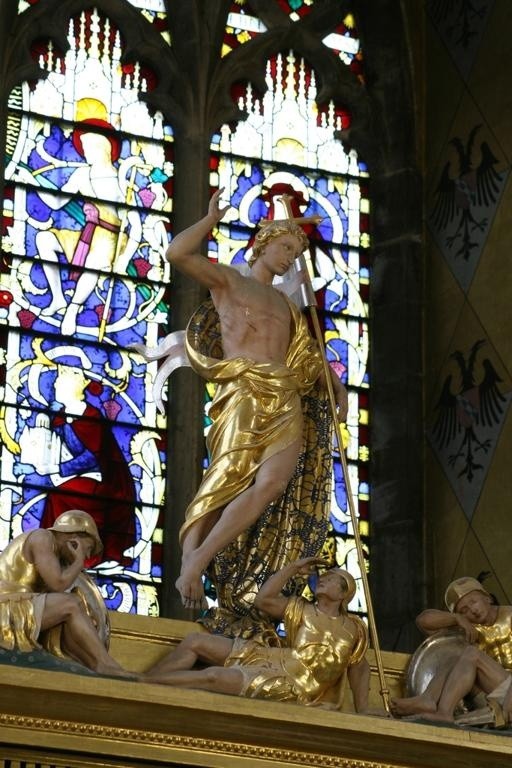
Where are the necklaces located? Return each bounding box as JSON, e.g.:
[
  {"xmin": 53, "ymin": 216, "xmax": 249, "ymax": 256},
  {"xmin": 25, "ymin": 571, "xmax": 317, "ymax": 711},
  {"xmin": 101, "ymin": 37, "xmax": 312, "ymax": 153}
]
[{"xmin": 486, "ymin": 606, "xmax": 500, "ymax": 627}]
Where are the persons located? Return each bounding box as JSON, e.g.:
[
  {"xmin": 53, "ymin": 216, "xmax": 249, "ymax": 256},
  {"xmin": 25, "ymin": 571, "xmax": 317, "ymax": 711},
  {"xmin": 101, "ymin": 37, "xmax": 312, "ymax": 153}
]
[
  {"xmin": 387, "ymin": 576, "xmax": 512, "ymax": 728},
  {"xmin": 163, "ymin": 185, "xmax": 349, "ymax": 609},
  {"xmin": 1, "ymin": 510, "xmax": 125, "ymax": 672},
  {"xmin": 95, "ymin": 557, "xmax": 386, "ymax": 716},
  {"xmin": 35, "ymin": 97, "xmax": 142, "ymax": 338}
]
